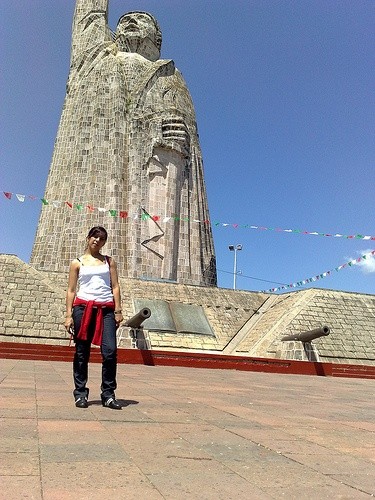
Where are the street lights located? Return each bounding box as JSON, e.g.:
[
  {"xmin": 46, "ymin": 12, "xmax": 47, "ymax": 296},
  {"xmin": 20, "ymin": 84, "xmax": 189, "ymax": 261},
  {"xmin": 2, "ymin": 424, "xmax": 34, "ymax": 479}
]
[{"xmin": 228, "ymin": 244, "xmax": 244, "ymax": 292}]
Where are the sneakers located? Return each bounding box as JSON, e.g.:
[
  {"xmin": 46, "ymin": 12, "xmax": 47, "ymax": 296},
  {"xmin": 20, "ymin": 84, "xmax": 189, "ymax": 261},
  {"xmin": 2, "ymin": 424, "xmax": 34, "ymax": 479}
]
[
  {"xmin": 102, "ymin": 396, "xmax": 122, "ymax": 409},
  {"xmin": 75, "ymin": 396, "xmax": 88, "ymax": 408}
]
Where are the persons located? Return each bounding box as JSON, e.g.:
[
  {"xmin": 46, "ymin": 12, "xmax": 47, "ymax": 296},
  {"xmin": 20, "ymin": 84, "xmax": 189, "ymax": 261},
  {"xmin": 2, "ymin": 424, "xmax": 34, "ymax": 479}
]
[
  {"xmin": 65, "ymin": 226, "xmax": 124, "ymax": 409},
  {"xmin": 30, "ymin": 0, "xmax": 217, "ymax": 286}
]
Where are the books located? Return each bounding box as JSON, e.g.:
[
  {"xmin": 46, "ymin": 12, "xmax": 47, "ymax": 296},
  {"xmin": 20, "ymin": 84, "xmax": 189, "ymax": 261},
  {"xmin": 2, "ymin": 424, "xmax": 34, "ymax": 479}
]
[{"xmin": 132, "ymin": 298, "xmax": 214, "ymax": 336}]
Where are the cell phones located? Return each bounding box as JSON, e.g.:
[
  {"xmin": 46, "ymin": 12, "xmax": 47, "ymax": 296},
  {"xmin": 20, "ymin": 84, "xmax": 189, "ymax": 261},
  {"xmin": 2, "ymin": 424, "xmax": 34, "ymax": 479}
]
[{"xmin": 69, "ymin": 327, "xmax": 74, "ymax": 335}]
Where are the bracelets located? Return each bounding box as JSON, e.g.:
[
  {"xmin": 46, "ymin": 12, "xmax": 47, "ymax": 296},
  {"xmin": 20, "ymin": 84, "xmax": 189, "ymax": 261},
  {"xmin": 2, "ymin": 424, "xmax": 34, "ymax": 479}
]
[{"xmin": 115, "ymin": 310, "xmax": 121, "ymax": 314}]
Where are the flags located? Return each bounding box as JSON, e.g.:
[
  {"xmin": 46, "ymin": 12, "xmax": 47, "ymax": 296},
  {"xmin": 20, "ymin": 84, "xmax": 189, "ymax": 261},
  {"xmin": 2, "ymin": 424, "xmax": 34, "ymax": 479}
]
[
  {"xmin": 4, "ymin": 192, "xmax": 160, "ymax": 222},
  {"xmin": 163, "ymin": 217, "xmax": 342, "ymax": 238},
  {"xmin": 343, "ymin": 234, "xmax": 375, "ymax": 241},
  {"xmin": 268, "ymin": 250, "xmax": 375, "ymax": 292}
]
